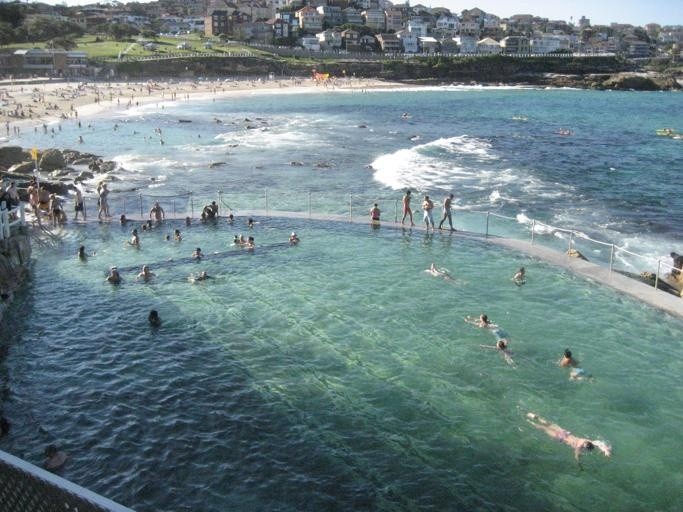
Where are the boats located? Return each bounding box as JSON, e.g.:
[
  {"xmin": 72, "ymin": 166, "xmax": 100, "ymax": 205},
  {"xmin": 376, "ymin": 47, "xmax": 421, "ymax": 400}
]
[
  {"xmin": 510, "ymin": 113, "xmax": 528, "ymax": 121},
  {"xmin": 553, "ymin": 130, "xmax": 571, "ymax": 136},
  {"xmin": 178, "ymin": 119, "xmax": 191, "ymax": 124},
  {"xmin": 654, "ymin": 128, "xmax": 680, "ymax": 137}
]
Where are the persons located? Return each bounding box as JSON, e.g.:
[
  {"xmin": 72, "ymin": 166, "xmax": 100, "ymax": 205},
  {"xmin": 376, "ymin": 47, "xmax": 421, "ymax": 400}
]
[
  {"xmin": 559, "ymin": 350, "xmax": 596, "ymax": 384},
  {"xmin": 0, "ymin": 79, "xmax": 256, "ymax": 284},
  {"xmin": 526, "ymin": 411, "xmax": 611, "ymax": 461},
  {"xmin": 402, "ymin": 192, "xmax": 413, "ymax": 225},
  {"xmin": 438, "ymin": 194, "xmax": 456, "ymax": 232},
  {"xmin": 422, "ymin": 196, "xmax": 434, "ymax": 232},
  {"xmin": 513, "ymin": 267, "xmax": 528, "ymax": 287},
  {"xmin": 425, "ymin": 260, "xmax": 454, "ymax": 282},
  {"xmin": 370, "ymin": 204, "xmax": 381, "ymax": 221},
  {"xmin": 463, "ymin": 313, "xmax": 506, "ymax": 339},
  {"xmin": 289, "ymin": 233, "xmax": 299, "ymax": 247},
  {"xmin": 148, "ymin": 309, "xmax": 162, "ymax": 328},
  {"xmin": 480, "ymin": 341, "xmax": 519, "ymax": 369}
]
